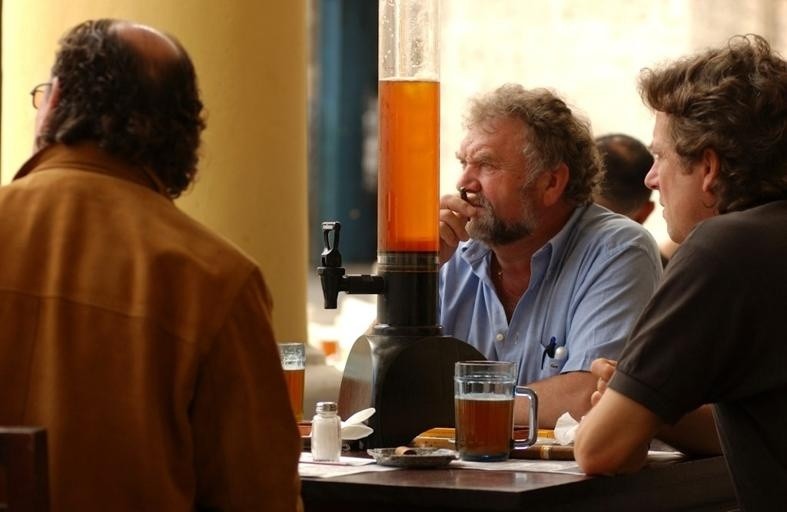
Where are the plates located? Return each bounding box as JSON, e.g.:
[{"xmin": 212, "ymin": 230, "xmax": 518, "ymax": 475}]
[{"xmin": 365, "ymin": 445, "xmax": 457, "ymax": 470}]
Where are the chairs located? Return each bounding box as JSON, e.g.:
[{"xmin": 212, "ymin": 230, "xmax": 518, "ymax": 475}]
[{"xmin": 0, "ymin": 425, "xmax": 51, "ymax": 511}]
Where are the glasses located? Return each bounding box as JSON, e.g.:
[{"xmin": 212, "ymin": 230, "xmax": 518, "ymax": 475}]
[{"xmin": 31, "ymin": 83, "xmax": 55, "ymax": 109}]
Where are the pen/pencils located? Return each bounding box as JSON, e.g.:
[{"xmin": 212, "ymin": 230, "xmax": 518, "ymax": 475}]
[
  {"xmin": 460, "ymin": 188, "xmax": 471, "ymax": 222},
  {"xmin": 541, "ymin": 336, "xmax": 556, "ymax": 370}
]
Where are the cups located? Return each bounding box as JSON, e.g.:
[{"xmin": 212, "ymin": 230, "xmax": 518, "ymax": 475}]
[
  {"xmin": 275, "ymin": 342, "xmax": 306, "ymax": 426},
  {"xmin": 453, "ymin": 358, "xmax": 539, "ymax": 464}
]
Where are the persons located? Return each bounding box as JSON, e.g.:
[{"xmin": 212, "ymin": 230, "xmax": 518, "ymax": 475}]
[
  {"xmin": 577, "ymin": 33, "xmax": 786, "ymax": 510},
  {"xmin": 0, "ymin": 20, "xmax": 307, "ymax": 511},
  {"xmin": 585, "ymin": 127, "xmax": 660, "ymax": 229},
  {"xmin": 403, "ymin": 82, "xmax": 672, "ymax": 444}
]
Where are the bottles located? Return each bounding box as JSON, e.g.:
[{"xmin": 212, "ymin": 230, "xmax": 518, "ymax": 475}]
[{"xmin": 310, "ymin": 400, "xmax": 343, "ymax": 465}]
